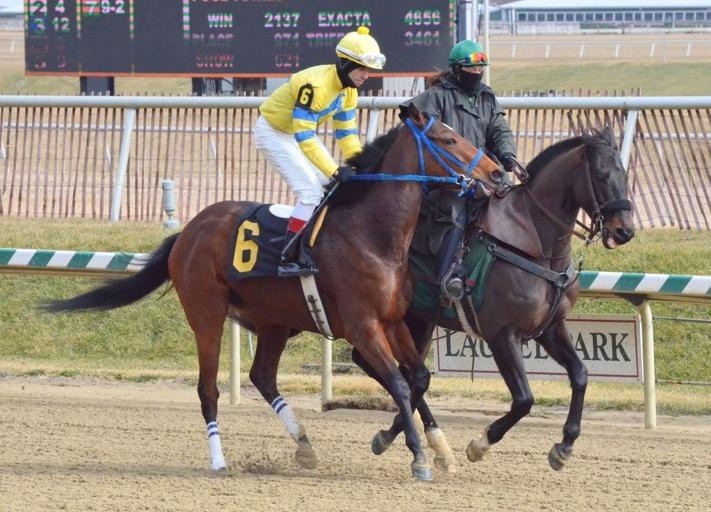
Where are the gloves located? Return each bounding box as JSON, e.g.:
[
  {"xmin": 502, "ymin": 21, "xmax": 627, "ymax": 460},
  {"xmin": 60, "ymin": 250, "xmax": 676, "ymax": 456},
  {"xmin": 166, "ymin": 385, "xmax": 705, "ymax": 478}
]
[
  {"xmin": 332, "ymin": 166, "xmax": 356, "ymax": 184},
  {"xmin": 498, "ymin": 152, "xmax": 516, "ymax": 172}
]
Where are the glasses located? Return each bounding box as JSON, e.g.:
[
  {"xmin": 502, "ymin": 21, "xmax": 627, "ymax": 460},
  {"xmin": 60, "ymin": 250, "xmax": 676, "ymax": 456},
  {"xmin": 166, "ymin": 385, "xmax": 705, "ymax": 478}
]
[
  {"xmin": 359, "ymin": 53, "xmax": 386, "ymax": 67},
  {"xmin": 458, "ymin": 52, "xmax": 487, "ymax": 64}
]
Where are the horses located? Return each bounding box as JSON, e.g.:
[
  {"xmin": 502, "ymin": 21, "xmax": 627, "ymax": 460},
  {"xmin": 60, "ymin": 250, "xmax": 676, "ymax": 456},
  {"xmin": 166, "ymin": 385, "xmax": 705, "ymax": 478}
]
[
  {"xmin": 350, "ymin": 121, "xmax": 636, "ymax": 476},
  {"xmin": 30, "ymin": 101, "xmax": 504, "ymax": 481}
]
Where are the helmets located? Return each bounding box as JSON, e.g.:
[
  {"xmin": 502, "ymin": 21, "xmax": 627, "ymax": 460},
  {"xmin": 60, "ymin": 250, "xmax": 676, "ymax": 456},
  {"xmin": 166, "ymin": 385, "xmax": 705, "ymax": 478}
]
[
  {"xmin": 447, "ymin": 40, "xmax": 490, "ymax": 67},
  {"xmin": 335, "ymin": 26, "xmax": 383, "ymax": 70}
]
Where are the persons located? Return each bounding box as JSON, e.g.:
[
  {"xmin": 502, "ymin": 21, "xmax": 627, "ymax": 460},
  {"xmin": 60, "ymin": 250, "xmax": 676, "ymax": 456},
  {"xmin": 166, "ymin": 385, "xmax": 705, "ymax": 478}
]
[
  {"xmin": 398, "ymin": 40, "xmax": 518, "ymax": 307},
  {"xmin": 254, "ymin": 26, "xmax": 386, "ymax": 278}
]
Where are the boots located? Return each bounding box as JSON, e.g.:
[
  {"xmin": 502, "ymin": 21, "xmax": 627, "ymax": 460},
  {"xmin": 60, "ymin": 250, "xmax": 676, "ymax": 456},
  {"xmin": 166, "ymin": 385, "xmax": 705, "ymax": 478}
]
[
  {"xmin": 277, "ymin": 216, "xmax": 318, "ymax": 278},
  {"xmin": 434, "ymin": 225, "xmax": 463, "ymax": 309}
]
